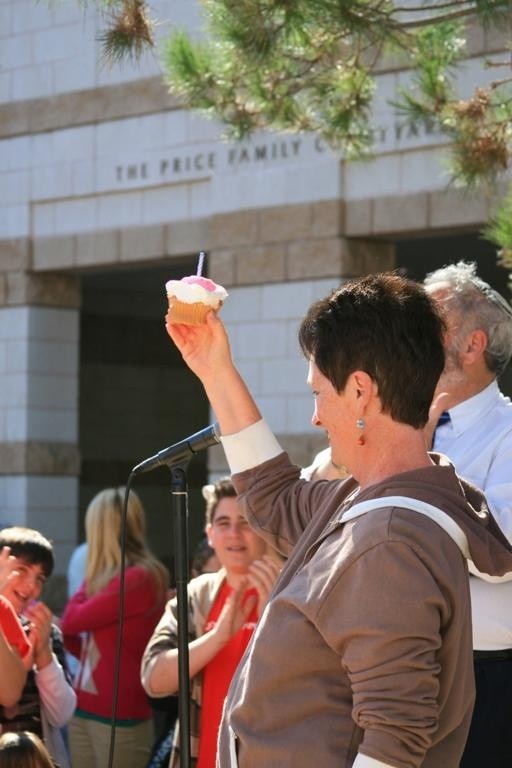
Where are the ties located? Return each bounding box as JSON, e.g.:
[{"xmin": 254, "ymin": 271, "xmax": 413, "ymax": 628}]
[{"xmin": 431, "ymin": 411, "xmax": 449, "ymax": 448}]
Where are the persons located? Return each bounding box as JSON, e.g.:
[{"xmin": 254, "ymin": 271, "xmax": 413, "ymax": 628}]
[
  {"xmin": 161, "ymin": 259, "xmax": 512, "ymax": 768},
  {"xmin": 135, "ymin": 477, "xmax": 288, "ymax": 767},
  {"xmin": 0, "ymin": 525, "xmax": 78, "ymax": 767},
  {"xmin": 164, "ymin": 273, "xmax": 511, "ymax": 768},
  {"xmin": 294, "ymin": 263, "xmax": 511, "ymax": 766},
  {"xmin": 62, "ymin": 488, "xmax": 173, "ymax": 768}
]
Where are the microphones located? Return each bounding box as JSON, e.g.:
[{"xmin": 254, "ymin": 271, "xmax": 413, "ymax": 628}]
[{"xmin": 134, "ymin": 420, "xmax": 223, "ymax": 474}]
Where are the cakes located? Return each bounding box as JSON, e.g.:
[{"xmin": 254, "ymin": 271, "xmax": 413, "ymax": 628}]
[{"xmin": 165, "ymin": 252, "xmax": 229, "ymax": 325}]
